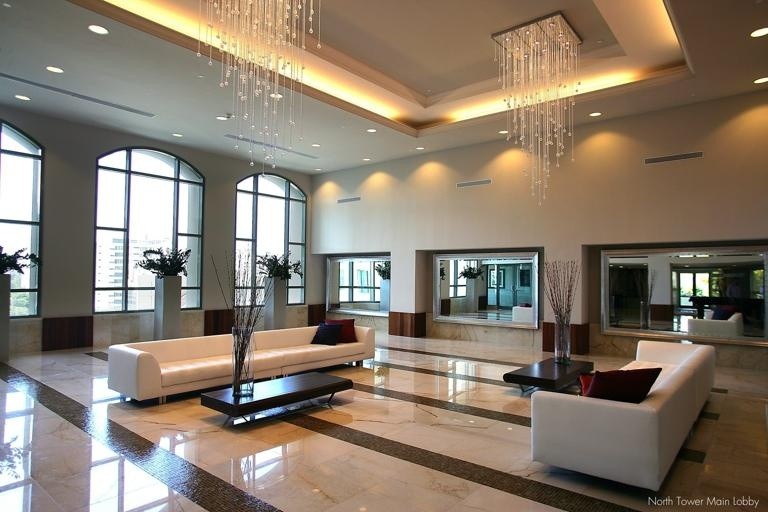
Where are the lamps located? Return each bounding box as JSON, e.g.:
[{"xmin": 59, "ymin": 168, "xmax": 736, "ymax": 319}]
[
  {"xmin": 487, "ymin": 11, "xmax": 584, "ymax": 209},
  {"xmin": 196, "ymin": 0, "xmax": 322, "ymax": 182}
]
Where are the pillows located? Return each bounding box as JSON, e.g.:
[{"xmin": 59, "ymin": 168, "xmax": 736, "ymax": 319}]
[
  {"xmin": 307, "ymin": 321, "xmax": 340, "ymax": 347},
  {"xmin": 710, "ymin": 302, "xmax": 738, "ymax": 322},
  {"xmin": 577, "ymin": 371, "xmax": 596, "ymax": 397},
  {"xmin": 325, "ymin": 318, "xmax": 359, "ymax": 343},
  {"xmin": 584, "ymin": 367, "xmax": 663, "ymax": 404}
]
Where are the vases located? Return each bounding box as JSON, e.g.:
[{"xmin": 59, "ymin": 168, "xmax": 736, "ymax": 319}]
[
  {"xmin": 639, "ymin": 301, "xmax": 652, "ymax": 331},
  {"xmin": 230, "ymin": 325, "xmax": 256, "ymax": 398},
  {"xmin": 553, "ymin": 316, "xmax": 572, "ymax": 366}
]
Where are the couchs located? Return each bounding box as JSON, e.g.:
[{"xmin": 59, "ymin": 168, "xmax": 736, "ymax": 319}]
[
  {"xmin": 526, "ymin": 338, "xmax": 718, "ymax": 492},
  {"xmin": 685, "ymin": 302, "xmax": 743, "ymax": 339},
  {"xmin": 510, "ymin": 302, "xmax": 534, "ymax": 324},
  {"xmin": 106, "ymin": 322, "xmax": 377, "ymax": 409}
]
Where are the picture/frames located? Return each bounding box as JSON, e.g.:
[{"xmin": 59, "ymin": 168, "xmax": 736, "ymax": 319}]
[{"xmin": 488, "ymin": 267, "xmax": 506, "ymax": 290}]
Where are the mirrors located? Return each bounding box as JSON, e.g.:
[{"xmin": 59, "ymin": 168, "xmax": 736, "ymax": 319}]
[
  {"xmin": 430, "ymin": 250, "xmax": 540, "ymax": 331},
  {"xmin": 324, "ymin": 255, "xmax": 391, "ymax": 318},
  {"xmin": 598, "ymin": 244, "xmax": 768, "ymax": 348}
]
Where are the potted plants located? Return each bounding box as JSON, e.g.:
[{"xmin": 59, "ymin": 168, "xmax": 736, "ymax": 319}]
[
  {"xmin": 455, "ymin": 262, "xmax": 487, "ymax": 313},
  {"xmin": 251, "ymin": 245, "xmax": 293, "ymax": 331},
  {"xmin": 130, "ymin": 243, "xmax": 194, "ymax": 343},
  {"xmin": 372, "ymin": 260, "xmax": 390, "ymax": 312},
  {"xmin": 0, "ymin": 242, "xmax": 41, "ymax": 368}
]
